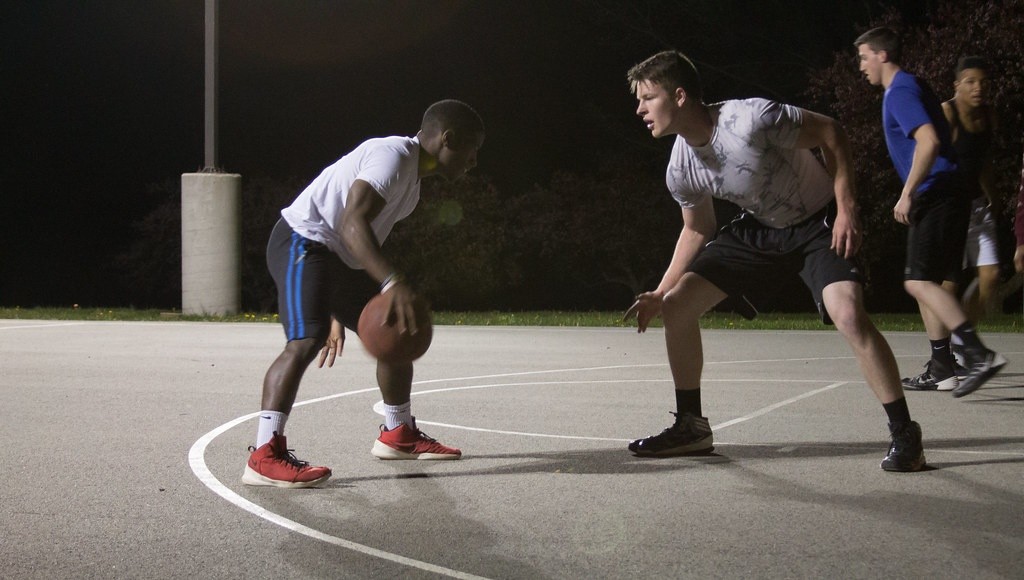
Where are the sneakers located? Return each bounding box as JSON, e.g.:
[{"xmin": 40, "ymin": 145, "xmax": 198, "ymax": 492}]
[
  {"xmin": 628, "ymin": 410, "xmax": 714, "ymax": 457},
  {"xmin": 900, "ymin": 361, "xmax": 959, "ymax": 391},
  {"xmin": 371, "ymin": 416, "xmax": 461, "ymax": 460},
  {"xmin": 241, "ymin": 431, "xmax": 332, "ymax": 489},
  {"xmin": 951, "ymin": 347, "xmax": 971, "ymax": 380},
  {"xmin": 954, "ymin": 348, "xmax": 1008, "ymax": 397},
  {"xmin": 881, "ymin": 421, "xmax": 926, "ymax": 473}
]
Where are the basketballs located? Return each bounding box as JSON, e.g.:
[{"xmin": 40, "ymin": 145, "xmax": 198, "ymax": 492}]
[{"xmin": 357, "ymin": 294, "xmax": 432, "ymax": 365}]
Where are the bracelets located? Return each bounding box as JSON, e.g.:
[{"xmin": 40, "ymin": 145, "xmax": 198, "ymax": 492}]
[{"xmin": 381, "ymin": 271, "xmax": 405, "ymax": 293}]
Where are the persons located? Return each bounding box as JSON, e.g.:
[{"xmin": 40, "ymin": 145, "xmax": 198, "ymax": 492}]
[
  {"xmin": 1014, "ymin": 155, "xmax": 1024, "ymax": 273},
  {"xmin": 854, "ymin": 27, "xmax": 1009, "ymax": 396},
  {"xmin": 940, "ymin": 55, "xmax": 1003, "ymax": 381},
  {"xmin": 243, "ymin": 100, "xmax": 485, "ymax": 487},
  {"xmin": 623, "ymin": 52, "xmax": 927, "ymax": 472}
]
